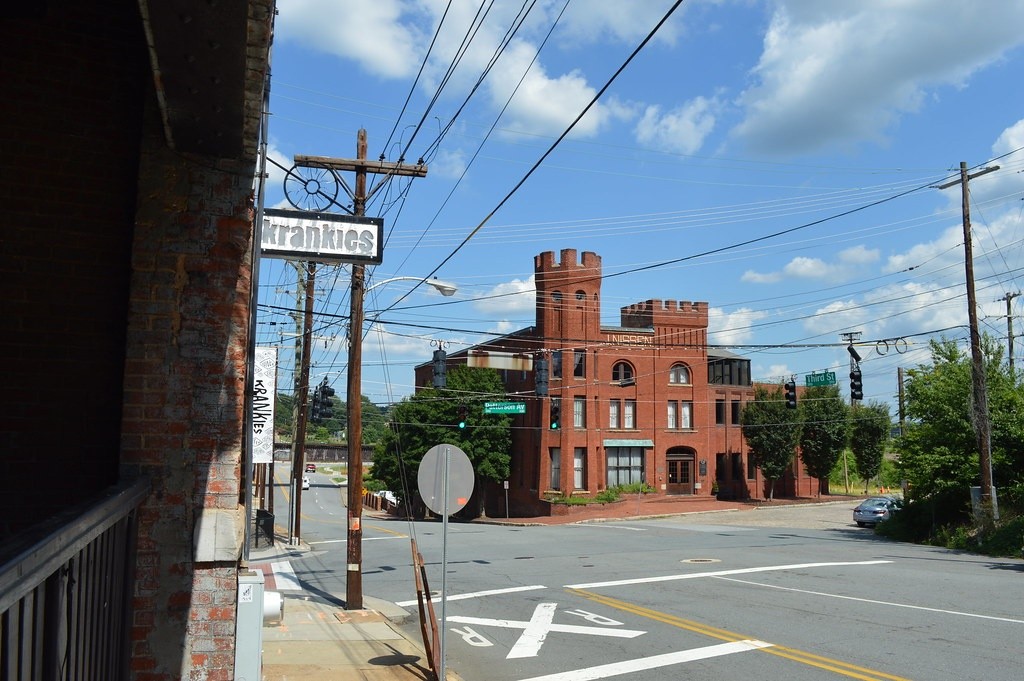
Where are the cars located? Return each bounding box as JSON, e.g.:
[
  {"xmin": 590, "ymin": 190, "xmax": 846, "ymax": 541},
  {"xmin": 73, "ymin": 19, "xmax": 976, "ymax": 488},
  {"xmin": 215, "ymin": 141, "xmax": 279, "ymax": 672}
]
[
  {"xmin": 302, "ymin": 476, "xmax": 310, "ymax": 490},
  {"xmin": 853, "ymin": 496, "xmax": 904, "ymax": 527},
  {"xmin": 374, "ymin": 491, "xmax": 393, "ymax": 497}
]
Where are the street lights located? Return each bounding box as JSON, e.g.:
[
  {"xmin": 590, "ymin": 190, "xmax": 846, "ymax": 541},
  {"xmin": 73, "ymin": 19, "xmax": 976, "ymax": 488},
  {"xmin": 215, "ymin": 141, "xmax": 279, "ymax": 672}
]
[{"xmin": 346, "ymin": 276, "xmax": 457, "ymax": 611}]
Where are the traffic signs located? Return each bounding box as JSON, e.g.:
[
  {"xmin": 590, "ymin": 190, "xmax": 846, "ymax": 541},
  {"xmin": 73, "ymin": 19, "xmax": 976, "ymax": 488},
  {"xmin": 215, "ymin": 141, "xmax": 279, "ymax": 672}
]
[{"xmin": 805, "ymin": 372, "xmax": 836, "ymax": 387}]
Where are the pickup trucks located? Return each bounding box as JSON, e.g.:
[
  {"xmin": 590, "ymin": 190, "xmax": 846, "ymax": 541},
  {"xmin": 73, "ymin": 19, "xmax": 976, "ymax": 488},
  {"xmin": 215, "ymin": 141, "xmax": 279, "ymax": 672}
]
[{"xmin": 305, "ymin": 464, "xmax": 316, "ymax": 472}]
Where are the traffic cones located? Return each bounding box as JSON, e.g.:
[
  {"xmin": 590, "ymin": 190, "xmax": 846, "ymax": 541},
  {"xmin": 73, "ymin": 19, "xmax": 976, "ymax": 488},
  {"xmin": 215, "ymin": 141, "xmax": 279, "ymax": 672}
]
[{"xmin": 881, "ymin": 486, "xmax": 885, "ymax": 493}]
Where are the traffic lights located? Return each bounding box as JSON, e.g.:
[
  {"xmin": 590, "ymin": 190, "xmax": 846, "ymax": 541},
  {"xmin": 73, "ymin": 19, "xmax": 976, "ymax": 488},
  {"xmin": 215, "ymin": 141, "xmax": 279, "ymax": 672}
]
[
  {"xmin": 784, "ymin": 382, "xmax": 797, "ymax": 409},
  {"xmin": 849, "ymin": 371, "xmax": 863, "ymax": 400},
  {"xmin": 312, "ymin": 397, "xmax": 322, "ymax": 423},
  {"xmin": 458, "ymin": 405, "xmax": 468, "ymax": 431},
  {"xmin": 319, "ymin": 386, "xmax": 335, "ymax": 418},
  {"xmin": 550, "ymin": 406, "xmax": 559, "ymax": 429}
]
